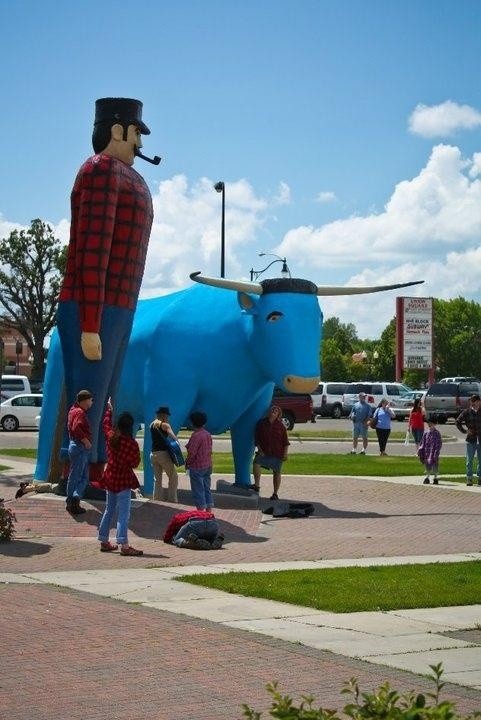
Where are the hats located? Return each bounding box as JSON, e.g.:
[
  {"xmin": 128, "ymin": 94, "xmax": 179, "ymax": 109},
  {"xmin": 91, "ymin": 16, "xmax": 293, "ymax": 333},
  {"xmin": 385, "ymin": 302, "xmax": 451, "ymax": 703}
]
[
  {"xmin": 359, "ymin": 392, "xmax": 367, "ymax": 397},
  {"xmin": 156, "ymin": 408, "xmax": 171, "ymax": 414}
]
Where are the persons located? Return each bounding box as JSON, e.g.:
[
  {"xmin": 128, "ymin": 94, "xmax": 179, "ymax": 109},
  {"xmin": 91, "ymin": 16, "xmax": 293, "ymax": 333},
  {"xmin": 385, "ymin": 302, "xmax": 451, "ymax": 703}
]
[
  {"xmin": 96, "ymin": 395, "xmax": 145, "ymax": 558},
  {"xmin": 370, "ymin": 398, "xmax": 397, "ymax": 457},
  {"xmin": 148, "ymin": 407, "xmax": 182, "ymax": 505},
  {"xmin": 347, "ymin": 391, "xmax": 373, "ymax": 455},
  {"xmin": 456, "ymin": 393, "xmax": 481, "ymax": 486},
  {"xmin": 185, "ymin": 408, "xmax": 215, "ymax": 515},
  {"xmin": 249, "ymin": 404, "xmax": 292, "ymax": 500},
  {"xmin": 417, "ymin": 419, "xmax": 443, "ymax": 485},
  {"xmin": 66, "ymin": 390, "xmax": 95, "ymax": 518},
  {"xmin": 164, "ymin": 511, "xmax": 226, "ymax": 551},
  {"xmin": 33, "ymin": 94, "xmax": 154, "ymax": 504}
]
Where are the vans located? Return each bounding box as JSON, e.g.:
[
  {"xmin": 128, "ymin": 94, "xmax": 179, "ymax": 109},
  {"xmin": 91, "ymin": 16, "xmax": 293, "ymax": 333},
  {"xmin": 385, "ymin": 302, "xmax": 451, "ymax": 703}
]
[{"xmin": 0, "ymin": 374, "xmax": 31, "ymax": 406}]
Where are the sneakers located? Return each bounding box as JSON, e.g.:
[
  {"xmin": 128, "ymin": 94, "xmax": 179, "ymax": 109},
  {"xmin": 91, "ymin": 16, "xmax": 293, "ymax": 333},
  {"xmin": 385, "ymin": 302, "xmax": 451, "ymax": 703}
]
[
  {"xmin": 467, "ymin": 476, "xmax": 473, "ymax": 486},
  {"xmin": 15, "ymin": 482, "xmax": 29, "ymax": 499},
  {"xmin": 247, "ymin": 484, "xmax": 261, "ymax": 492},
  {"xmin": 66, "ymin": 495, "xmax": 86, "ymax": 514},
  {"xmin": 270, "ymin": 494, "xmax": 278, "ymax": 500},
  {"xmin": 185, "ymin": 532, "xmax": 225, "ymax": 550},
  {"xmin": 101, "ymin": 542, "xmax": 118, "ymax": 551},
  {"xmin": 424, "ymin": 478, "xmax": 438, "ymax": 484},
  {"xmin": 120, "ymin": 547, "xmax": 143, "ymax": 555},
  {"xmin": 351, "ymin": 452, "xmax": 365, "ymax": 455}
]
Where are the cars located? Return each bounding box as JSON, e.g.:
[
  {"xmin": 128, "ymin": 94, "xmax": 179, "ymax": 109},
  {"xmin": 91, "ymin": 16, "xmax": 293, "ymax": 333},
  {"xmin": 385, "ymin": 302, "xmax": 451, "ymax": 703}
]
[{"xmin": 2, "ymin": 393, "xmax": 43, "ymax": 431}]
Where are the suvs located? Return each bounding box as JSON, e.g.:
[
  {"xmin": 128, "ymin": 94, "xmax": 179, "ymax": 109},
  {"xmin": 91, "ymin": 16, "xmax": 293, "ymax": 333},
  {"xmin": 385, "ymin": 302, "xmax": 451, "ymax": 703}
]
[{"xmin": 263, "ymin": 375, "xmax": 480, "ymax": 432}]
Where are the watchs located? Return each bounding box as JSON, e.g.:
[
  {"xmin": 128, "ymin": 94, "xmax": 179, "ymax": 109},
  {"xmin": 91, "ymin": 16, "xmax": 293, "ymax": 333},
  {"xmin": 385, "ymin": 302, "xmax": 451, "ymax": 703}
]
[{"xmin": 406, "ymin": 398, "xmax": 426, "ymax": 447}]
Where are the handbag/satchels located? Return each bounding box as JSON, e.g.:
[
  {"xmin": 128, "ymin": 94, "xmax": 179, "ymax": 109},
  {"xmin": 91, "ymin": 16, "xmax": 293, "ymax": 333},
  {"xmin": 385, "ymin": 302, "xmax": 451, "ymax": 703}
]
[{"xmin": 368, "ymin": 418, "xmax": 377, "ymax": 429}]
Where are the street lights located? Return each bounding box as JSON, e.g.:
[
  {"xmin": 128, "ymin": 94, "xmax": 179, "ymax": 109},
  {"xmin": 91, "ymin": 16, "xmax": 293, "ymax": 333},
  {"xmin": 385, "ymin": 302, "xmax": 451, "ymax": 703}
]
[
  {"xmin": 361, "ymin": 351, "xmax": 382, "ymax": 381},
  {"xmin": 212, "ymin": 180, "xmax": 225, "ymax": 278},
  {"xmin": 249, "ymin": 256, "xmax": 287, "ymax": 282},
  {"xmin": 256, "ymin": 252, "xmax": 292, "ymax": 278}
]
